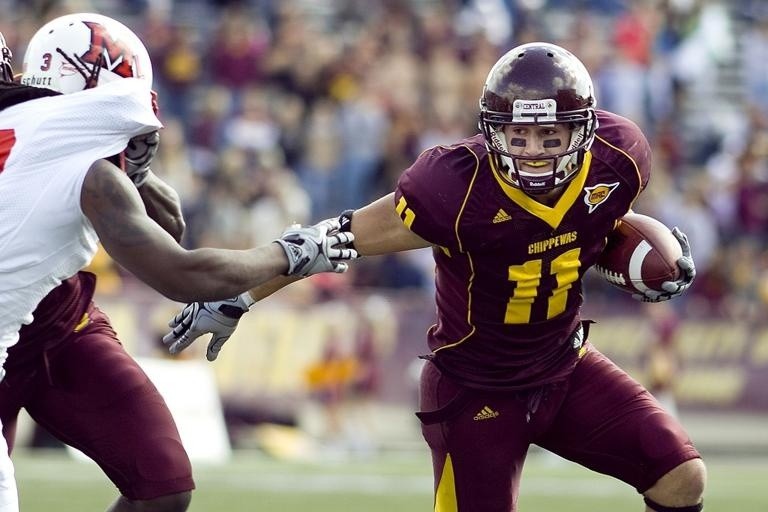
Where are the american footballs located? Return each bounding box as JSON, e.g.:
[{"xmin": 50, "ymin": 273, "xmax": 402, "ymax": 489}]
[{"xmin": 592, "ymin": 213, "xmax": 683, "ymax": 295}]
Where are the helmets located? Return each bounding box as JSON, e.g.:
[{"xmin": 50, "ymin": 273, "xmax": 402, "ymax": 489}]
[
  {"xmin": 21, "ymin": 12, "xmax": 153, "ymax": 95},
  {"xmin": 479, "ymin": 41, "xmax": 598, "ymax": 196}
]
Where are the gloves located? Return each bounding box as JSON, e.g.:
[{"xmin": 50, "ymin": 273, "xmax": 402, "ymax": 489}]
[
  {"xmin": 630, "ymin": 226, "xmax": 696, "ymax": 303},
  {"xmin": 274, "ymin": 218, "xmax": 359, "ymax": 276},
  {"xmin": 164, "ymin": 291, "xmax": 249, "ymax": 360},
  {"xmin": 124, "ymin": 93, "xmax": 159, "ymax": 185}
]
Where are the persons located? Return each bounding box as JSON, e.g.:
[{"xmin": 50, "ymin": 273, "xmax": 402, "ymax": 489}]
[
  {"xmin": 0, "ymin": 11, "xmax": 358, "ymax": 512},
  {"xmin": 0, "ymin": 29, "xmax": 187, "ymax": 512},
  {"xmin": 162, "ymin": 41, "xmax": 708, "ymax": 512},
  {"xmin": 2, "ymin": 1, "xmax": 766, "ymax": 308}
]
